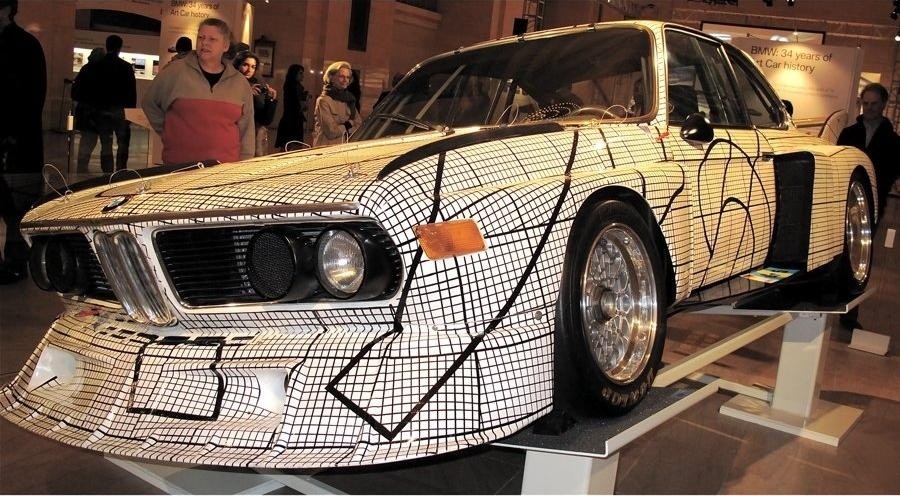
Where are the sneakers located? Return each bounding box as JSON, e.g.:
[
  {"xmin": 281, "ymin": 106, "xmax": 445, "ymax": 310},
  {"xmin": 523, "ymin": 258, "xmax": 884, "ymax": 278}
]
[{"xmin": 1, "ymin": 267, "xmax": 27, "ymax": 284}]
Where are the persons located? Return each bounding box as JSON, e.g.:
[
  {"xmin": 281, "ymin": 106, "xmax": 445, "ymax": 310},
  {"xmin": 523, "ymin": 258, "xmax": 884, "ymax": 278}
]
[
  {"xmin": 161, "ymin": 37, "xmax": 197, "ymax": 66},
  {"xmin": 140, "ymin": 18, "xmax": 255, "ymax": 171},
  {"xmin": 835, "ymin": 82, "xmax": 900, "ymax": 332},
  {"xmin": 70, "ymin": 48, "xmax": 105, "ymax": 172},
  {"xmin": 430, "ymin": 82, "xmax": 492, "ymax": 125},
  {"xmin": 345, "ymin": 68, "xmax": 361, "ymax": 114},
  {"xmin": 0, "ymin": 0, "xmax": 47, "ymax": 285},
  {"xmin": 312, "ymin": 59, "xmax": 365, "ymax": 147},
  {"xmin": 274, "ymin": 63, "xmax": 312, "ymax": 152},
  {"xmin": 88, "ymin": 34, "xmax": 137, "ymax": 174},
  {"xmin": 226, "ymin": 42, "xmax": 250, "ymax": 60},
  {"xmin": 233, "ymin": 50, "xmax": 277, "ymax": 159}
]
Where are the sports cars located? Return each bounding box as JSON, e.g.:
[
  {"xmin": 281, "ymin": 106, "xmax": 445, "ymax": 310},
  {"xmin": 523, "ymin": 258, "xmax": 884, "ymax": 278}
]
[{"xmin": 2, "ymin": 18, "xmax": 885, "ymax": 473}]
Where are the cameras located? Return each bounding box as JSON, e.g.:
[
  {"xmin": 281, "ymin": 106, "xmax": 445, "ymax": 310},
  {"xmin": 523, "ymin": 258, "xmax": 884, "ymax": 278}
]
[{"xmin": 256, "ymin": 85, "xmax": 268, "ymax": 95}]
